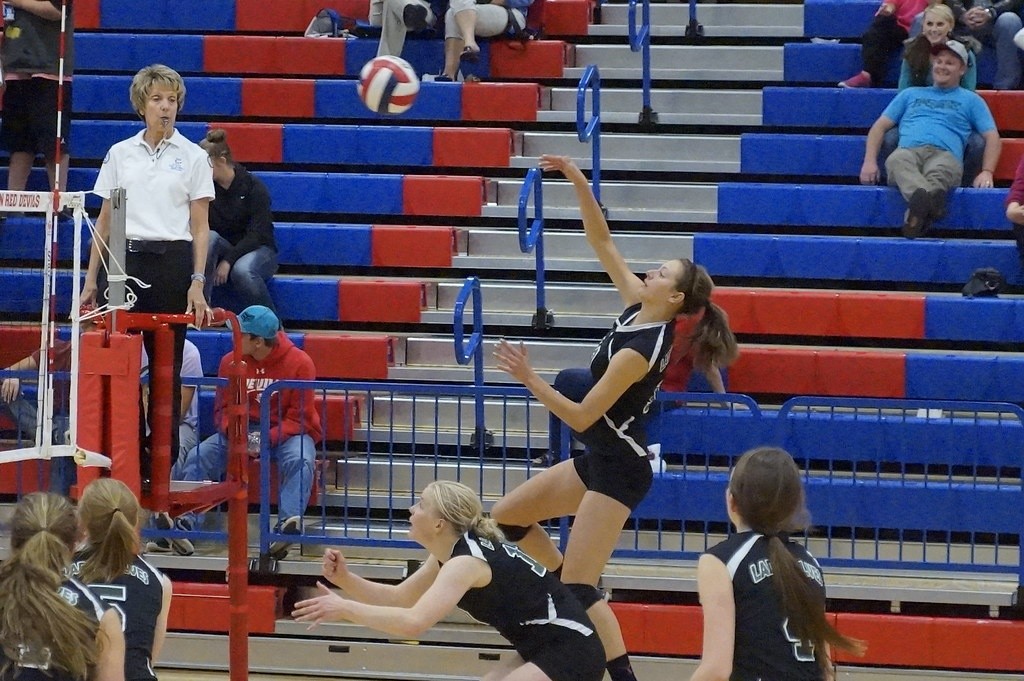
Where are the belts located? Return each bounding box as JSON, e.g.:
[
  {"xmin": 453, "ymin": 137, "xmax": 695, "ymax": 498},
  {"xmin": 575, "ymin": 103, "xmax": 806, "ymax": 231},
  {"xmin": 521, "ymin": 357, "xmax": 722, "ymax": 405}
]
[{"xmin": 125, "ymin": 241, "xmax": 189, "ymax": 253}]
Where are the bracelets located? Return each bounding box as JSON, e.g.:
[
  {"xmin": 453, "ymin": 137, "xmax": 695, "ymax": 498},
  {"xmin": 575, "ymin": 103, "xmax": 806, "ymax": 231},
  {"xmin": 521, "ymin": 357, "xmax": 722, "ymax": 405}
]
[{"xmin": 191, "ymin": 273, "xmax": 206, "ymax": 284}]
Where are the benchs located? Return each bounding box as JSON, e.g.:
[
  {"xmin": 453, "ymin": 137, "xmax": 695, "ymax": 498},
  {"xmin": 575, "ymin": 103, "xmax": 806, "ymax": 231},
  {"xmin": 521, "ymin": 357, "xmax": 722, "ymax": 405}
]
[{"xmin": 0, "ymin": 0, "xmax": 1024, "ymax": 675}]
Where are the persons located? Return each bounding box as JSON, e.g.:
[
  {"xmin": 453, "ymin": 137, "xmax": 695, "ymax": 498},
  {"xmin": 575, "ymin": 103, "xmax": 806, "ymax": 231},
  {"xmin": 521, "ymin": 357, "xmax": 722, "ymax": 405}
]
[
  {"xmin": 68, "ymin": 62, "xmax": 215, "ymax": 492},
  {"xmin": 491, "ymin": 153, "xmax": 738, "ymax": 681},
  {"xmin": 153, "ymin": 304, "xmax": 325, "ymax": 561},
  {"xmin": 72, "ymin": 476, "xmax": 171, "ymax": 681},
  {"xmin": 0, "ymin": 491, "xmax": 126, "ymax": 681},
  {"xmin": 877, "ymin": 3, "xmax": 986, "ymax": 187},
  {"xmin": 0, "ymin": 0, "xmax": 76, "ymax": 222},
  {"xmin": 141, "ymin": 331, "xmax": 204, "ymax": 553},
  {"xmin": 1004, "ymin": 150, "xmax": 1024, "ymax": 257},
  {"xmin": 435, "ymin": 0, "xmax": 535, "ymax": 83},
  {"xmin": 532, "ymin": 306, "xmax": 737, "ymax": 468},
  {"xmin": 859, "ymin": 40, "xmax": 1002, "ymax": 240},
  {"xmin": 836, "ymin": 0, "xmax": 942, "ymax": 89},
  {"xmin": 291, "ymin": 479, "xmax": 606, "ymax": 681},
  {"xmin": 909, "ymin": 0, "xmax": 1024, "ymax": 89},
  {"xmin": 685, "ymin": 445, "xmax": 866, "ymax": 681},
  {"xmin": 368, "ymin": 0, "xmax": 449, "ymax": 57},
  {"xmin": 195, "ymin": 130, "xmax": 284, "ymax": 334},
  {"xmin": 0, "ymin": 338, "xmax": 77, "ymax": 495}
]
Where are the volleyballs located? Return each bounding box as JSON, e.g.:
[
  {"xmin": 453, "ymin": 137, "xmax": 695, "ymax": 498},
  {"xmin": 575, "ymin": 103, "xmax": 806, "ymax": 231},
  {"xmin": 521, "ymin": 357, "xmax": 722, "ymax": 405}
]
[{"xmin": 356, "ymin": 55, "xmax": 420, "ymax": 117}]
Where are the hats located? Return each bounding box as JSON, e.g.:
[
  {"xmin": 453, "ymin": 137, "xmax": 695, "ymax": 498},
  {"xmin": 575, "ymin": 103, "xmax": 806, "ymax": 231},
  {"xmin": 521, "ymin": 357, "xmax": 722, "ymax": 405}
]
[
  {"xmin": 931, "ymin": 40, "xmax": 968, "ymax": 64},
  {"xmin": 226, "ymin": 305, "xmax": 279, "ymax": 339}
]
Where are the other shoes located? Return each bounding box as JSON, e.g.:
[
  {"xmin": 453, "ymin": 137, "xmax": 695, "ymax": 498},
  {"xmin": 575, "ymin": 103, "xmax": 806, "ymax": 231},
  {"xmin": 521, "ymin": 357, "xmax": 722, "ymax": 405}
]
[
  {"xmin": 268, "ymin": 514, "xmax": 300, "ymax": 559},
  {"xmin": 55, "ymin": 428, "xmax": 70, "ymax": 444},
  {"xmin": 402, "ymin": 2, "xmax": 437, "ymax": 38},
  {"xmin": 146, "ymin": 537, "xmax": 172, "ymax": 552},
  {"xmin": 154, "ymin": 512, "xmax": 194, "ymax": 556},
  {"xmin": 532, "ymin": 450, "xmax": 560, "ymax": 466},
  {"xmin": 903, "ymin": 188, "xmax": 947, "ymax": 240}
]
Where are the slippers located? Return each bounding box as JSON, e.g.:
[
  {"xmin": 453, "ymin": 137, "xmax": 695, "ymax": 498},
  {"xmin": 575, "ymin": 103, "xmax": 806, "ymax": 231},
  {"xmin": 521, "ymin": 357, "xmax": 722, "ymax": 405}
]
[
  {"xmin": 435, "ymin": 73, "xmax": 452, "ymax": 82},
  {"xmin": 461, "ymin": 45, "xmax": 480, "ymax": 63}
]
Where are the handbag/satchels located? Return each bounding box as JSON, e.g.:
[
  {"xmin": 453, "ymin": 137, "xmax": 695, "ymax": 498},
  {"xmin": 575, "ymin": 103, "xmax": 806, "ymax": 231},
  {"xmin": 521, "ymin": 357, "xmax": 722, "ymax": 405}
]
[
  {"xmin": 304, "ymin": 9, "xmax": 357, "ymax": 40},
  {"xmin": 961, "ymin": 266, "xmax": 1002, "ymax": 297}
]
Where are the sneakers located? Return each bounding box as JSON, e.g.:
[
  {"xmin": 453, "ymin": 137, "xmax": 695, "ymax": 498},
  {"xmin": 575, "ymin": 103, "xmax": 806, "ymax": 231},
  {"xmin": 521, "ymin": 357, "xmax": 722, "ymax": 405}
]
[{"xmin": 837, "ymin": 72, "xmax": 869, "ymax": 88}]
